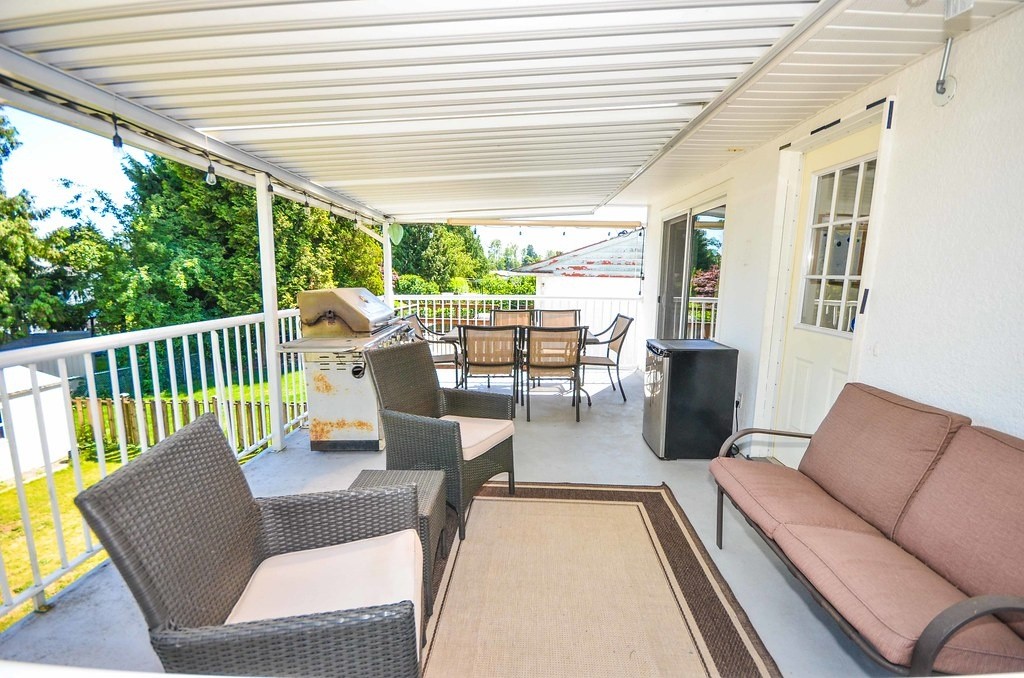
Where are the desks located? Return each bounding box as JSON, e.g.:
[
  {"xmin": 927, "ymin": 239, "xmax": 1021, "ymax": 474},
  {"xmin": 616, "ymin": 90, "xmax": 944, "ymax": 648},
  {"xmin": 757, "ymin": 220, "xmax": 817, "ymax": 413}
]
[{"xmin": 440, "ymin": 327, "xmax": 599, "ymax": 407}]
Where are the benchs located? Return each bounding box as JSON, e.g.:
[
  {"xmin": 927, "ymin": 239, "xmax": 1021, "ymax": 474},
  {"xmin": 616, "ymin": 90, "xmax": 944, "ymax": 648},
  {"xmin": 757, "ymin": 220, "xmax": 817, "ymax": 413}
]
[{"xmin": 708, "ymin": 383, "xmax": 1024, "ymax": 678}]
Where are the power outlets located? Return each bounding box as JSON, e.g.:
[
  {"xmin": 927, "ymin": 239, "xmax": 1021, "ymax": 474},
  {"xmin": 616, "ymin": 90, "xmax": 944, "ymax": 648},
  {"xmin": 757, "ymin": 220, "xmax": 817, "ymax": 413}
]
[{"xmin": 736, "ymin": 392, "xmax": 743, "ymax": 409}]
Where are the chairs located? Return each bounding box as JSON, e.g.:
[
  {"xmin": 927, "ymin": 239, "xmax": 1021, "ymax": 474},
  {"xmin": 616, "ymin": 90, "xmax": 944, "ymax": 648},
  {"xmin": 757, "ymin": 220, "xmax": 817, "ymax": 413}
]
[
  {"xmin": 73, "ymin": 411, "xmax": 433, "ymax": 678},
  {"xmin": 401, "ymin": 313, "xmax": 463, "ymax": 388},
  {"xmin": 577, "ymin": 312, "xmax": 634, "ymax": 403},
  {"xmin": 455, "ymin": 309, "xmax": 589, "ymax": 422},
  {"xmin": 364, "ymin": 340, "xmax": 515, "ymax": 541}
]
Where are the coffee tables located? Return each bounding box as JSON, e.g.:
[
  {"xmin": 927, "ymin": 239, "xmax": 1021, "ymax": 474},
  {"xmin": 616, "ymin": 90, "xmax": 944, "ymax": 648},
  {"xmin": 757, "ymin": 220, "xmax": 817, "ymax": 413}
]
[{"xmin": 348, "ymin": 470, "xmax": 447, "ymax": 616}]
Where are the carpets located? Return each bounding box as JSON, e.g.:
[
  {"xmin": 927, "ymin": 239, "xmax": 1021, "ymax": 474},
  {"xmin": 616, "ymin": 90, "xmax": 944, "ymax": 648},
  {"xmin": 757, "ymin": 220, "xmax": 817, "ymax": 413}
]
[{"xmin": 420, "ymin": 480, "xmax": 784, "ymax": 678}]
